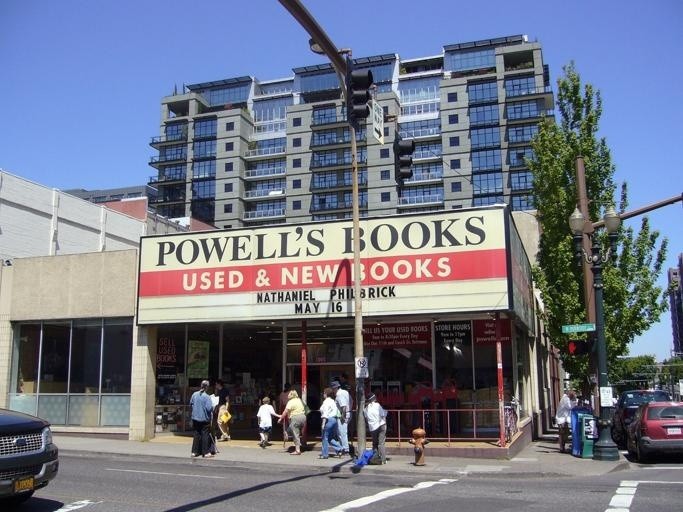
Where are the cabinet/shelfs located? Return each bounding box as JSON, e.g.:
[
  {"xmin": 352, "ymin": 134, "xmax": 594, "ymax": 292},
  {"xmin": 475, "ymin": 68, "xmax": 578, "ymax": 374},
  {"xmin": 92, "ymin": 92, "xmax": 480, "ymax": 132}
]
[{"xmin": 155, "ymin": 386, "xmax": 251, "ymax": 432}]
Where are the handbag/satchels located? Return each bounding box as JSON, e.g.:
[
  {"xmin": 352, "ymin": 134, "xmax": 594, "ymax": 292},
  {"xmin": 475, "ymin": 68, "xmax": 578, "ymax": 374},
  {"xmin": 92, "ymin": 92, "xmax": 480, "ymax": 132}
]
[
  {"xmin": 368, "ymin": 451, "xmax": 382, "ymax": 465},
  {"xmin": 299, "ymin": 398, "xmax": 311, "ymax": 415}
]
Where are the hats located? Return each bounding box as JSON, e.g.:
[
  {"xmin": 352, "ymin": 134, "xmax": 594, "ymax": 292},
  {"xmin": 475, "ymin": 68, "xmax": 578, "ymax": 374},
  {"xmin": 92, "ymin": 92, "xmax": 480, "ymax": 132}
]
[
  {"xmin": 365, "ymin": 390, "xmax": 376, "ymax": 402},
  {"xmin": 328, "ymin": 380, "xmax": 341, "ymax": 389}
]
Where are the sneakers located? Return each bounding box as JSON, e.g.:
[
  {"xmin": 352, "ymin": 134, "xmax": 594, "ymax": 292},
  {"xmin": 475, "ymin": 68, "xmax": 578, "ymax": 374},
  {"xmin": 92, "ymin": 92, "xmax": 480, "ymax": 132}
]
[{"xmin": 256, "ymin": 438, "xmax": 353, "ymax": 459}]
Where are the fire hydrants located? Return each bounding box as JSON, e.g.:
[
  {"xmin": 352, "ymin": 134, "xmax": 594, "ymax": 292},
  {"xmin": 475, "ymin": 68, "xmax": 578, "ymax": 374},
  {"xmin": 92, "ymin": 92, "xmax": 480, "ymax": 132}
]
[{"xmin": 409, "ymin": 429, "xmax": 427, "ymax": 467}]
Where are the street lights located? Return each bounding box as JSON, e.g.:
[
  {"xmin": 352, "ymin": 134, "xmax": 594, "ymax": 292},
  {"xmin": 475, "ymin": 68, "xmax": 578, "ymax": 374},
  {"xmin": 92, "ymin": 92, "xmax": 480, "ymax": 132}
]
[{"xmin": 569, "ymin": 203, "xmax": 621, "ymax": 460}]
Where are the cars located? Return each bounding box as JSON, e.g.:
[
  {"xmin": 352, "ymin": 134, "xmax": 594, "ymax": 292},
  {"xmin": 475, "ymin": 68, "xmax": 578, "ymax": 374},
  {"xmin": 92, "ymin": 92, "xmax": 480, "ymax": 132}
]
[
  {"xmin": 1, "ymin": 408, "xmax": 59, "ymax": 504},
  {"xmin": 612, "ymin": 390, "xmax": 683, "ymax": 461}
]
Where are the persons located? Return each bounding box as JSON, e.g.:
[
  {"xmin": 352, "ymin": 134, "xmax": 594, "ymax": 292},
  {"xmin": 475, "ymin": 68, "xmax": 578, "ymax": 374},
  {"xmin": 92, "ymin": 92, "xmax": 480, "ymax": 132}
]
[
  {"xmin": 338, "ymin": 372, "xmax": 353, "ymax": 395},
  {"xmin": 279, "ymin": 383, "xmax": 291, "ymax": 439},
  {"xmin": 276, "ymin": 389, "xmax": 307, "ymax": 455},
  {"xmin": 361, "ymin": 392, "xmax": 390, "ymax": 465},
  {"xmin": 317, "ymin": 387, "xmax": 345, "ymax": 459},
  {"xmin": 554, "ymin": 390, "xmax": 577, "ymax": 453},
  {"xmin": 211, "ymin": 378, "xmax": 232, "ymax": 442},
  {"xmin": 328, "ymin": 380, "xmax": 354, "ymax": 456},
  {"xmin": 188, "ymin": 379, "xmax": 216, "ymax": 458},
  {"xmin": 255, "ymin": 396, "xmax": 281, "ymax": 447},
  {"xmin": 257, "ymin": 386, "xmax": 276, "ymax": 441}
]
